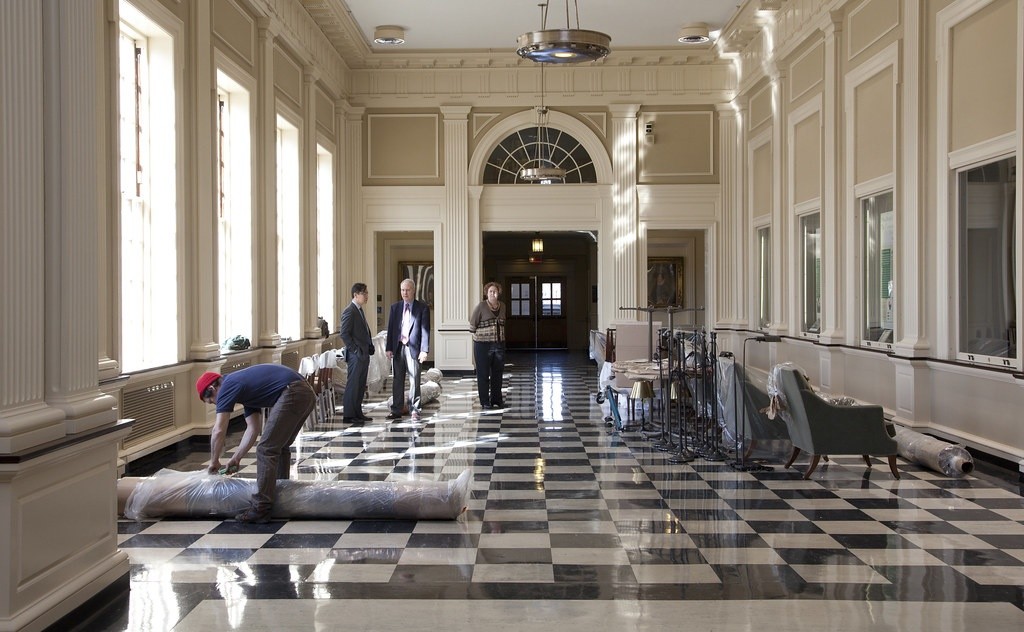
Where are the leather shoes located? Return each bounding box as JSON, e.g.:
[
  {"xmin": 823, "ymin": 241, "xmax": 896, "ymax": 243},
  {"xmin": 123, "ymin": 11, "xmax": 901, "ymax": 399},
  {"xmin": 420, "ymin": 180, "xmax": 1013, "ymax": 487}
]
[
  {"xmin": 358, "ymin": 415, "xmax": 373, "ymax": 421},
  {"xmin": 343, "ymin": 417, "xmax": 364, "ymax": 424}
]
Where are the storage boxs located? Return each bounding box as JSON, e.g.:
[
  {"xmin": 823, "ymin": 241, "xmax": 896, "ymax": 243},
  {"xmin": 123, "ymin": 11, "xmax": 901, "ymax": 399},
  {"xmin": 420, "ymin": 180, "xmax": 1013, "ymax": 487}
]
[{"xmin": 615, "ymin": 322, "xmax": 662, "ymax": 389}]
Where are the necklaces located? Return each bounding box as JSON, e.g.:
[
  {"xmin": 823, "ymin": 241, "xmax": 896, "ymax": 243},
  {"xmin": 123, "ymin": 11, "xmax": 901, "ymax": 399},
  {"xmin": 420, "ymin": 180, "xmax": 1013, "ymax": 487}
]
[{"xmin": 488, "ymin": 300, "xmax": 499, "ymax": 310}]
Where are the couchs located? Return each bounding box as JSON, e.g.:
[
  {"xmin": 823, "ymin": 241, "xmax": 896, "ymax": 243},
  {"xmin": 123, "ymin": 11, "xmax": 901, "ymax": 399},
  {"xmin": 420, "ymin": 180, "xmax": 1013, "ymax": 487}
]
[
  {"xmin": 772, "ymin": 365, "xmax": 901, "ymax": 479},
  {"xmin": 717, "ymin": 354, "xmax": 830, "ymax": 462}
]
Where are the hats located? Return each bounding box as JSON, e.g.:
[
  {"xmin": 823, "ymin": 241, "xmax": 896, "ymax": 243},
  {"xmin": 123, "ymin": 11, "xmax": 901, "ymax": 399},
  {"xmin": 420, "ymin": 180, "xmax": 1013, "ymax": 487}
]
[{"xmin": 196, "ymin": 371, "xmax": 221, "ymax": 400}]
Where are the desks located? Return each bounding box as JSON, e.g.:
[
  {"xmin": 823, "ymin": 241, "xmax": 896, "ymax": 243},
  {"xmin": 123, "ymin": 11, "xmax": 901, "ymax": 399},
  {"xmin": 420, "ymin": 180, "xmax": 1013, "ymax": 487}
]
[{"xmin": 611, "ymin": 306, "xmax": 712, "ymax": 441}]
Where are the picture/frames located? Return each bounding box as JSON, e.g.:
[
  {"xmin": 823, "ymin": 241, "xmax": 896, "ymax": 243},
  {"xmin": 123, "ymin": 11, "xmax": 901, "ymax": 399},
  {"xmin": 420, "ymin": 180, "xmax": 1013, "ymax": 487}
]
[
  {"xmin": 397, "ymin": 260, "xmax": 434, "ymax": 311},
  {"xmin": 647, "ymin": 255, "xmax": 684, "ymax": 309}
]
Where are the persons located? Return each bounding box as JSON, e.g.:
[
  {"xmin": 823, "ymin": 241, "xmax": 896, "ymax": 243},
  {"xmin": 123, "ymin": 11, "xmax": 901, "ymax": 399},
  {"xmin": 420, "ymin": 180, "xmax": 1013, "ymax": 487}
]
[
  {"xmin": 469, "ymin": 282, "xmax": 512, "ymax": 409},
  {"xmin": 340, "ymin": 283, "xmax": 375, "ymax": 424},
  {"xmin": 384, "ymin": 278, "xmax": 431, "ymax": 421},
  {"xmin": 196, "ymin": 363, "xmax": 317, "ymax": 523}
]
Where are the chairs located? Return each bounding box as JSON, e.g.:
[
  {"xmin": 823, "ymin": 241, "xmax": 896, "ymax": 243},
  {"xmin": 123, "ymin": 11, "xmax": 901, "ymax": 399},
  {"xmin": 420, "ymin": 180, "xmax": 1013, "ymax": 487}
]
[{"xmin": 262, "ymin": 331, "xmax": 390, "ymax": 430}]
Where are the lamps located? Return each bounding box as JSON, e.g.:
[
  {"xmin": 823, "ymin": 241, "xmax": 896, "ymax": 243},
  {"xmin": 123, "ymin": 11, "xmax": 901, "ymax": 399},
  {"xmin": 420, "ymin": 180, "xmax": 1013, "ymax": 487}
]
[
  {"xmin": 629, "ymin": 380, "xmax": 657, "ymax": 433},
  {"xmin": 531, "ymin": 232, "xmax": 543, "ymax": 254},
  {"xmin": 671, "ymin": 381, "xmax": 693, "ymax": 436},
  {"xmin": 519, "ymin": 4, "xmax": 567, "ymax": 181},
  {"xmin": 516, "ymin": 0, "xmax": 612, "ymax": 63}
]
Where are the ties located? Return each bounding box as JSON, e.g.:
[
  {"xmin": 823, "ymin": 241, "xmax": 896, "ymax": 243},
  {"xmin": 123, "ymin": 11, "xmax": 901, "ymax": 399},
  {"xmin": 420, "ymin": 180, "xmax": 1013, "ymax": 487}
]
[
  {"xmin": 400, "ymin": 303, "xmax": 410, "ymax": 345},
  {"xmin": 359, "ymin": 307, "xmax": 370, "ymax": 334}
]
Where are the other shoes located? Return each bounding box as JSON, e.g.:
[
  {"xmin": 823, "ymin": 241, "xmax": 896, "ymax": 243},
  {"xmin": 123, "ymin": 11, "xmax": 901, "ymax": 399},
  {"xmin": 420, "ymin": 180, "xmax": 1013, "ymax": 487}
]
[
  {"xmin": 493, "ymin": 403, "xmax": 506, "ymax": 409},
  {"xmin": 411, "ymin": 413, "xmax": 420, "ymax": 421},
  {"xmin": 482, "ymin": 402, "xmax": 493, "ymax": 409},
  {"xmin": 385, "ymin": 413, "xmax": 402, "ymax": 419},
  {"xmin": 234, "ymin": 508, "xmax": 271, "ymax": 524}
]
